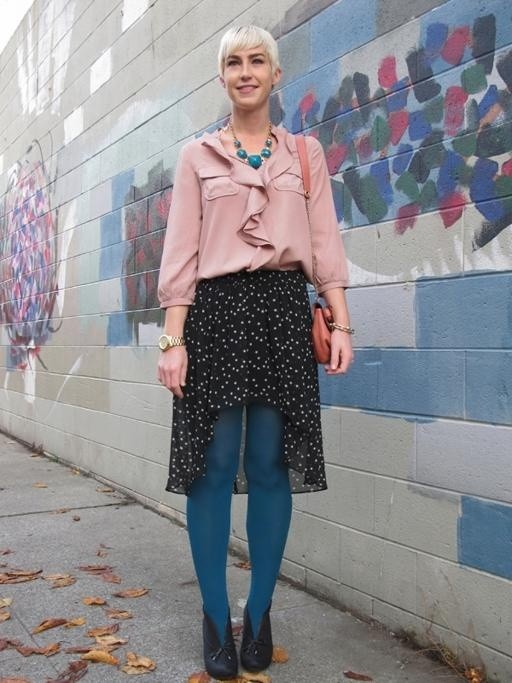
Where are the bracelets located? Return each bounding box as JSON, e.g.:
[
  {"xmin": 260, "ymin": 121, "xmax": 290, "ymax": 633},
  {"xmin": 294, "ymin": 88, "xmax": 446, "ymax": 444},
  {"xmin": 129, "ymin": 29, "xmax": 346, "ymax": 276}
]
[{"xmin": 328, "ymin": 321, "xmax": 355, "ymax": 335}]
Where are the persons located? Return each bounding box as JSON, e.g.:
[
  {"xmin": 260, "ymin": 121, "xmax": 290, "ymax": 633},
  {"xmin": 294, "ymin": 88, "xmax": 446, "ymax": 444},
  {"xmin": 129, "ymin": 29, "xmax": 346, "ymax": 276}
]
[{"xmin": 153, "ymin": 21, "xmax": 357, "ymax": 681}]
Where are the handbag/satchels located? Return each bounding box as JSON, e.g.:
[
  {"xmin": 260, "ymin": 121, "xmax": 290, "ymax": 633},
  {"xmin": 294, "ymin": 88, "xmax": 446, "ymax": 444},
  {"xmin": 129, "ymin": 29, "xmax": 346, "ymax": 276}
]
[{"xmin": 310, "ymin": 302, "xmax": 334, "ymax": 364}]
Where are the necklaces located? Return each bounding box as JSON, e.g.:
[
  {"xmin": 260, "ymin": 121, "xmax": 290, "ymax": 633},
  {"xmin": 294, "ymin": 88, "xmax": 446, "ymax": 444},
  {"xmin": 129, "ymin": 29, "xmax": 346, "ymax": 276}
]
[{"xmin": 228, "ymin": 116, "xmax": 273, "ymax": 169}]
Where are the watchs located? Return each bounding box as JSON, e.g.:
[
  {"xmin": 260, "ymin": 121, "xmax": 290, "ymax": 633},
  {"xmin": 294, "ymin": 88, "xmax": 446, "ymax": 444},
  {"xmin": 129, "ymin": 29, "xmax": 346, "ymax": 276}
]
[{"xmin": 156, "ymin": 333, "xmax": 189, "ymax": 352}]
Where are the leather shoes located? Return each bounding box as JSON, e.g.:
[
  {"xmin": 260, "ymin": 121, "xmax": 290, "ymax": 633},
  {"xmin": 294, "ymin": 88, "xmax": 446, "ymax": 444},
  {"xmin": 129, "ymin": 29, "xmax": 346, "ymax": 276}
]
[{"xmin": 203, "ymin": 602, "xmax": 273, "ymax": 680}]
